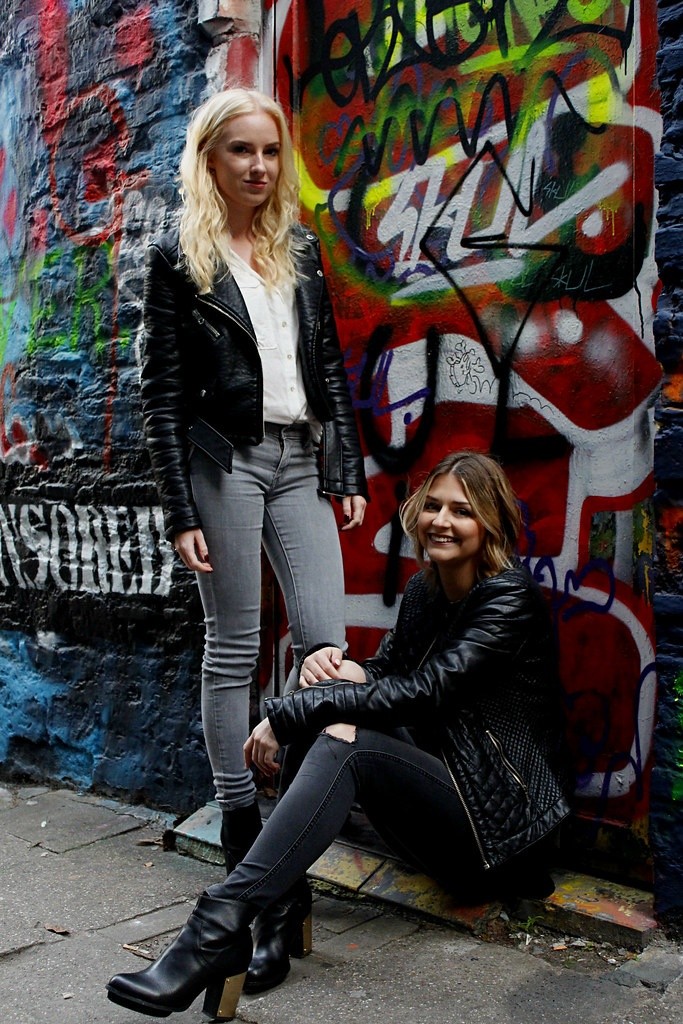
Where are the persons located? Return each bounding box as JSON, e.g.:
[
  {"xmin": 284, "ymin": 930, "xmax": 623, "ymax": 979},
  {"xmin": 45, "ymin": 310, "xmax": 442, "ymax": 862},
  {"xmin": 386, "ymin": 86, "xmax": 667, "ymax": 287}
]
[
  {"xmin": 138, "ymin": 90, "xmax": 369, "ymax": 882},
  {"xmin": 105, "ymin": 451, "xmax": 567, "ymax": 1022}
]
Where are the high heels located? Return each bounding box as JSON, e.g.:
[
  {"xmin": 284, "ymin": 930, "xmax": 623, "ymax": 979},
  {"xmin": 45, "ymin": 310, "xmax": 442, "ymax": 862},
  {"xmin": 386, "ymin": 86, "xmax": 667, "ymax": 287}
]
[
  {"xmin": 105, "ymin": 892, "xmax": 254, "ymax": 1022},
  {"xmin": 243, "ymin": 878, "xmax": 312, "ymax": 994}
]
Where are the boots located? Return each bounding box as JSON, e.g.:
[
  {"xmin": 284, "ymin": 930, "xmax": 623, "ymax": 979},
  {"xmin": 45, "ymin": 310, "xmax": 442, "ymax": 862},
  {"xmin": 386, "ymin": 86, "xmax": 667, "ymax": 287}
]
[
  {"xmin": 217, "ymin": 804, "xmax": 263, "ymax": 873},
  {"xmin": 276, "ymin": 742, "xmax": 312, "ymax": 808}
]
